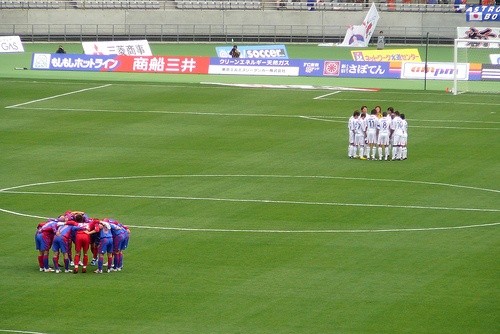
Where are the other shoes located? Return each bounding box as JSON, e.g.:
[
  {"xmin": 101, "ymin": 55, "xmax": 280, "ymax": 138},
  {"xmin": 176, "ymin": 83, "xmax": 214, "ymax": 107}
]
[
  {"xmin": 106, "ymin": 269, "xmax": 110, "ymax": 273},
  {"xmin": 70, "ymin": 261, "xmax": 74, "ymax": 266},
  {"xmin": 73, "ymin": 268, "xmax": 79, "ymax": 273},
  {"xmin": 79, "ymin": 261, "xmax": 83, "ymax": 266},
  {"xmin": 45, "ymin": 268, "xmax": 55, "ymax": 272},
  {"xmin": 48, "ymin": 265, "xmax": 54, "ymax": 268},
  {"xmin": 56, "ymin": 269, "xmax": 62, "ymax": 273},
  {"xmin": 58, "ymin": 264, "xmax": 63, "ymax": 267},
  {"xmin": 65, "ymin": 269, "xmax": 72, "ymax": 272},
  {"xmin": 91, "ymin": 259, "xmax": 96, "ymax": 265},
  {"xmin": 350, "ymin": 155, "xmax": 407, "ymax": 161},
  {"xmin": 40, "ymin": 267, "xmax": 45, "ymax": 271},
  {"xmin": 103, "ymin": 261, "xmax": 124, "ymax": 271},
  {"xmin": 82, "ymin": 268, "xmax": 86, "ymax": 273},
  {"xmin": 93, "ymin": 269, "xmax": 103, "ymax": 273}
]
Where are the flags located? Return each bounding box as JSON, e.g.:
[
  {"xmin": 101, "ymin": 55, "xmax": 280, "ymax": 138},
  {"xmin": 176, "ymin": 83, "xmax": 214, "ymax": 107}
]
[{"xmin": 361, "ymin": 2, "xmax": 380, "ymax": 44}]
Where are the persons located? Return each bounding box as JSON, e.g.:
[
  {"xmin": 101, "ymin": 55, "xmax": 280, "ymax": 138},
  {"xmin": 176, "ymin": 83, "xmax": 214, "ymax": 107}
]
[
  {"xmin": 57, "ymin": 45, "xmax": 66, "ymax": 53},
  {"xmin": 229, "ymin": 45, "xmax": 240, "ymax": 58},
  {"xmin": 347, "ymin": 105, "xmax": 408, "ymax": 161},
  {"xmin": 378, "ymin": 31, "xmax": 386, "ymax": 45},
  {"xmin": 35, "ymin": 211, "xmax": 130, "ymax": 273}
]
